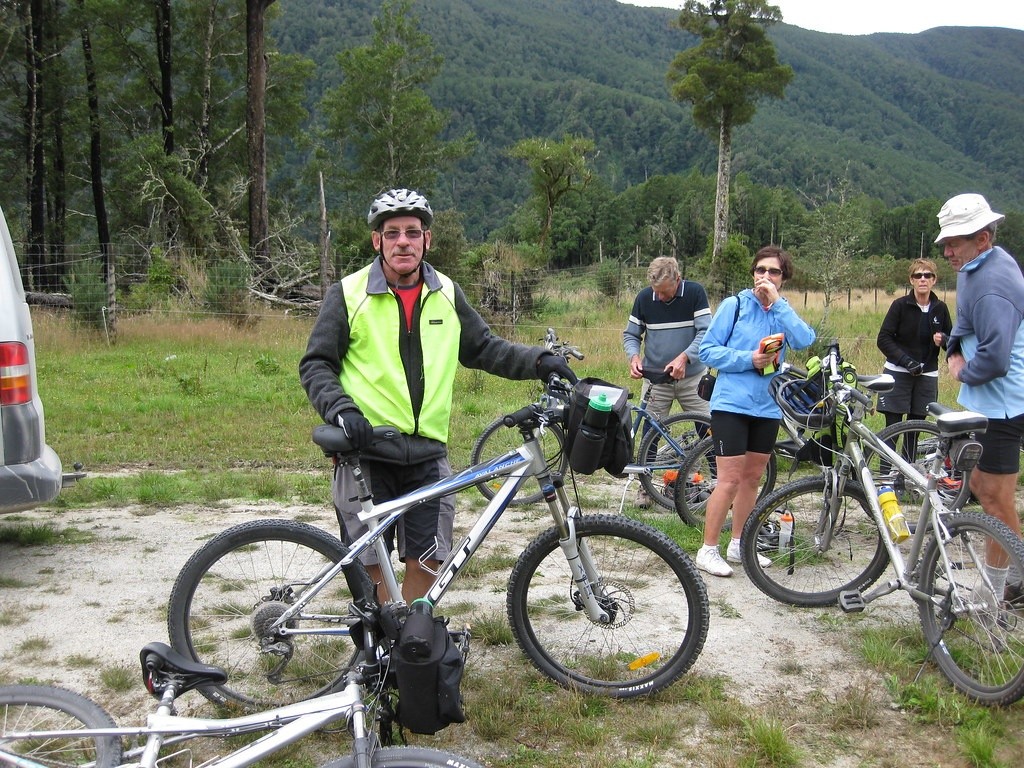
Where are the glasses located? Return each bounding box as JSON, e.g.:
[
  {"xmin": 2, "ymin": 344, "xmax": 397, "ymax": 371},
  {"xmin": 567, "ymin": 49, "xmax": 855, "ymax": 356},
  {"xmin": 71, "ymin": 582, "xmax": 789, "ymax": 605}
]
[
  {"xmin": 753, "ymin": 267, "xmax": 783, "ymax": 277},
  {"xmin": 910, "ymin": 273, "xmax": 936, "ymax": 279},
  {"xmin": 381, "ymin": 229, "xmax": 424, "ymax": 239}
]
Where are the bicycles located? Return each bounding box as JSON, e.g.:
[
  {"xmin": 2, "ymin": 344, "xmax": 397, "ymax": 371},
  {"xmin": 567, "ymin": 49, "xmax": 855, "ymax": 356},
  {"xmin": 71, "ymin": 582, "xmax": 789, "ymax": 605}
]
[
  {"xmin": 674, "ymin": 375, "xmax": 945, "ymax": 541},
  {"xmin": 739, "ymin": 346, "xmax": 1022, "ymax": 701},
  {"xmin": 0, "ymin": 618, "xmax": 478, "ymax": 768},
  {"xmin": 160, "ymin": 333, "xmax": 709, "ymax": 700},
  {"xmin": 475, "ymin": 322, "xmax": 720, "ymax": 510}
]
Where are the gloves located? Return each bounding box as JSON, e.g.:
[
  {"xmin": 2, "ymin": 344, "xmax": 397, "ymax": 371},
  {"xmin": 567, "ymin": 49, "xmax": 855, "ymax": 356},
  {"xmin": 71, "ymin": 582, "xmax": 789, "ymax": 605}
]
[
  {"xmin": 537, "ymin": 354, "xmax": 578, "ymax": 385},
  {"xmin": 335, "ymin": 407, "xmax": 374, "ymax": 451}
]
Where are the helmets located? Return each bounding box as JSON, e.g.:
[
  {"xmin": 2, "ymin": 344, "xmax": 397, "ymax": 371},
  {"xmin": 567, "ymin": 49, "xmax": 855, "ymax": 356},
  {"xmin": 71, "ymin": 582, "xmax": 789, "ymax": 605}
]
[
  {"xmin": 769, "ymin": 370, "xmax": 837, "ymax": 429},
  {"xmin": 367, "ymin": 187, "xmax": 433, "ymax": 231}
]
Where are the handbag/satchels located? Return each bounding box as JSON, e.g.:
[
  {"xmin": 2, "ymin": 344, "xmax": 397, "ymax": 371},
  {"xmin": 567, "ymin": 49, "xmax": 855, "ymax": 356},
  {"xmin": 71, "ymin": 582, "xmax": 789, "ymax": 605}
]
[{"xmin": 696, "ymin": 370, "xmax": 716, "ymax": 401}]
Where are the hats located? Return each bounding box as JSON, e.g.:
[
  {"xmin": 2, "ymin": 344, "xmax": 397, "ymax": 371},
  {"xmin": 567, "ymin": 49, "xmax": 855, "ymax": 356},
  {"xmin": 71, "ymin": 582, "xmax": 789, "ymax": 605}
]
[{"xmin": 933, "ymin": 192, "xmax": 1006, "ymax": 246}]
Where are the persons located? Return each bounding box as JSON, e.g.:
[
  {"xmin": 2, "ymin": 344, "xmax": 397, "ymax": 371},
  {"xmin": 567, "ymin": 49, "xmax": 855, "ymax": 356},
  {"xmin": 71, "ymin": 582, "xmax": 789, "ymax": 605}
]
[
  {"xmin": 299, "ymin": 190, "xmax": 578, "ymax": 656},
  {"xmin": 874, "ymin": 259, "xmax": 955, "ymax": 484},
  {"xmin": 621, "ymin": 257, "xmax": 712, "ymax": 508},
  {"xmin": 934, "ymin": 194, "xmax": 1024, "ymax": 614},
  {"xmin": 695, "ymin": 247, "xmax": 815, "ymax": 577}
]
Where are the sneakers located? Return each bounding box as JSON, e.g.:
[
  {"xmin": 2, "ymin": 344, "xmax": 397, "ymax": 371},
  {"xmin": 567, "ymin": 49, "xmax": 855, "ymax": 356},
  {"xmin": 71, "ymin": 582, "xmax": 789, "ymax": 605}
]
[
  {"xmin": 696, "ymin": 547, "xmax": 734, "ymax": 576},
  {"xmin": 726, "ymin": 538, "xmax": 773, "ymax": 567}
]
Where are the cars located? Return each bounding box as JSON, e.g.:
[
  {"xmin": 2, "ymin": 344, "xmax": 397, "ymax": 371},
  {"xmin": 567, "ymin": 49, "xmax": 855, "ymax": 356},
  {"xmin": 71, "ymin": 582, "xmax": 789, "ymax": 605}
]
[{"xmin": 0, "ymin": 207, "xmax": 71, "ymax": 515}]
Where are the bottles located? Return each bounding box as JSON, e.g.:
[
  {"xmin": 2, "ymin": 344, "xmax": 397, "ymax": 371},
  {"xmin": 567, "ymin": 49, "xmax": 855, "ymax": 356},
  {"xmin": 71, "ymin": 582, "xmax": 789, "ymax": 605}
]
[
  {"xmin": 877, "ymin": 486, "xmax": 909, "ymax": 543},
  {"xmin": 779, "ymin": 512, "xmax": 796, "ymax": 553},
  {"xmin": 408, "ymin": 598, "xmax": 434, "ymax": 618},
  {"xmin": 583, "ymin": 394, "xmax": 612, "ymax": 440}
]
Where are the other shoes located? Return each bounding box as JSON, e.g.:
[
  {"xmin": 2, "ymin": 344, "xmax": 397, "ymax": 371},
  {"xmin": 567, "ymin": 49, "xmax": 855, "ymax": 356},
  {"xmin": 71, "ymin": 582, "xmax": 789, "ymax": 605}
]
[
  {"xmin": 636, "ymin": 484, "xmax": 652, "ymax": 509},
  {"xmin": 1003, "ymin": 579, "xmax": 1023, "ymax": 606}
]
